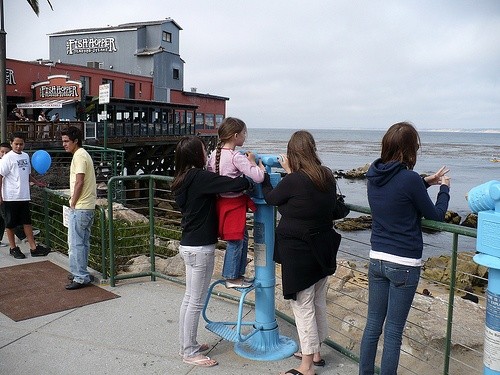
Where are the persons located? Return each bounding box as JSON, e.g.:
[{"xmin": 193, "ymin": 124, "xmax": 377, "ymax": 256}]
[
  {"xmin": 0, "ymin": 131, "xmax": 52, "ymax": 259},
  {"xmin": 8, "ymin": 107, "xmax": 59, "ymax": 121},
  {"xmin": 62, "ymin": 126, "xmax": 97, "ymax": 289},
  {"xmin": 170, "ymin": 135, "xmax": 254, "ymax": 368},
  {"xmin": 257, "ymin": 130, "xmax": 342, "ymax": 375},
  {"xmin": 206, "ymin": 117, "xmax": 264, "ymax": 289},
  {"xmin": 357, "ymin": 121, "xmax": 450, "ymax": 375}
]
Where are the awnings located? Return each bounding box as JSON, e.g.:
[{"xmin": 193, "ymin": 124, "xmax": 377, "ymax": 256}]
[{"xmin": 17, "ymin": 99, "xmax": 75, "ymax": 108}]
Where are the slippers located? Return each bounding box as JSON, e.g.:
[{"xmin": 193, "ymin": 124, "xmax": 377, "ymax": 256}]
[
  {"xmin": 183, "ymin": 356, "xmax": 218, "ymax": 367},
  {"xmin": 293, "ymin": 352, "xmax": 325, "ymax": 366},
  {"xmin": 179, "ymin": 343, "xmax": 203, "ymax": 357},
  {"xmin": 285, "ymin": 369, "xmax": 318, "ymax": 375}
]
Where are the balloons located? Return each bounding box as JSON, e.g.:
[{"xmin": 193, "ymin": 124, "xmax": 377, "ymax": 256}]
[{"xmin": 31, "ymin": 150, "xmax": 51, "ymax": 175}]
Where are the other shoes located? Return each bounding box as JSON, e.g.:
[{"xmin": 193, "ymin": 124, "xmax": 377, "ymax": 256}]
[
  {"xmin": 65, "ymin": 281, "xmax": 90, "ymax": 289},
  {"xmin": 225, "ymin": 281, "xmax": 253, "ymax": 289},
  {"xmin": 240, "ymin": 277, "xmax": 254, "ymax": 282},
  {"xmin": 67, "ymin": 275, "xmax": 94, "ymax": 282}
]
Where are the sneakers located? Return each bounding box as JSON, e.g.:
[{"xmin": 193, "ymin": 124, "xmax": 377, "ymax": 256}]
[
  {"xmin": 0, "ymin": 240, "xmax": 8, "ymax": 247},
  {"xmin": 30, "ymin": 244, "xmax": 51, "ymax": 256},
  {"xmin": 22, "ymin": 230, "xmax": 40, "ymax": 243},
  {"xmin": 10, "ymin": 245, "xmax": 25, "ymax": 259}
]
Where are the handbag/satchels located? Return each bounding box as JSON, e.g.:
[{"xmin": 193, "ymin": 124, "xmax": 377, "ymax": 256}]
[{"xmin": 333, "ymin": 195, "xmax": 349, "ymax": 220}]
